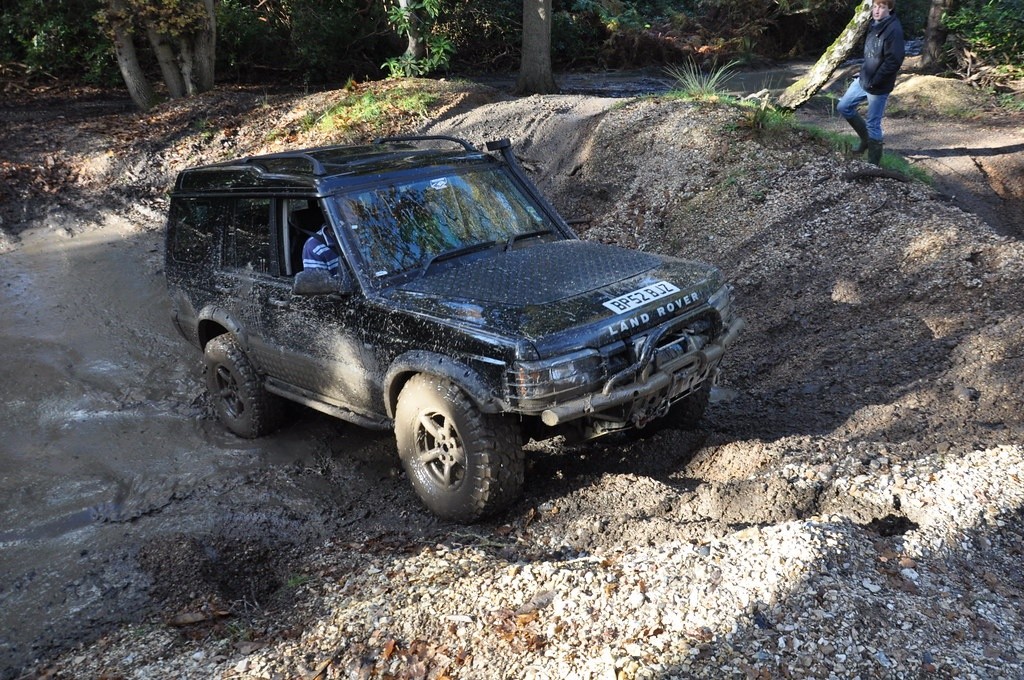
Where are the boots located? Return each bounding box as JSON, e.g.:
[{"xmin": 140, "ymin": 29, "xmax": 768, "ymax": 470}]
[
  {"xmin": 866, "ymin": 141, "xmax": 884, "ymax": 167},
  {"xmin": 851, "ymin": 128, "xmax": 870, "ymax": 154}
]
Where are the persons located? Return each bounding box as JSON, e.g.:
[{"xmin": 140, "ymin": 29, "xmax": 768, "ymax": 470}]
[
  {"xmin": 836, "ymin": 0, "xmax": 906, "ymax": 165},
  {"xmin": 304, "ymin": 198, "xmax": 382, "ymax": 282}
]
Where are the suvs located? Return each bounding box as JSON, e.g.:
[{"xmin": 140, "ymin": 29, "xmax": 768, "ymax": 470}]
[{"xmin": 163, "ymin": 132, "xmax": 746, "ymax": 527}]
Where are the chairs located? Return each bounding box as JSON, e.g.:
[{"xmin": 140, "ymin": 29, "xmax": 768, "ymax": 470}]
[
  {"xmin": 289, "ymin": 206, "xmax": 327, "ymax": 276},
  {"xmin": 234, "ymin": 202, "xmax": 297, "ymax": 253}
]
